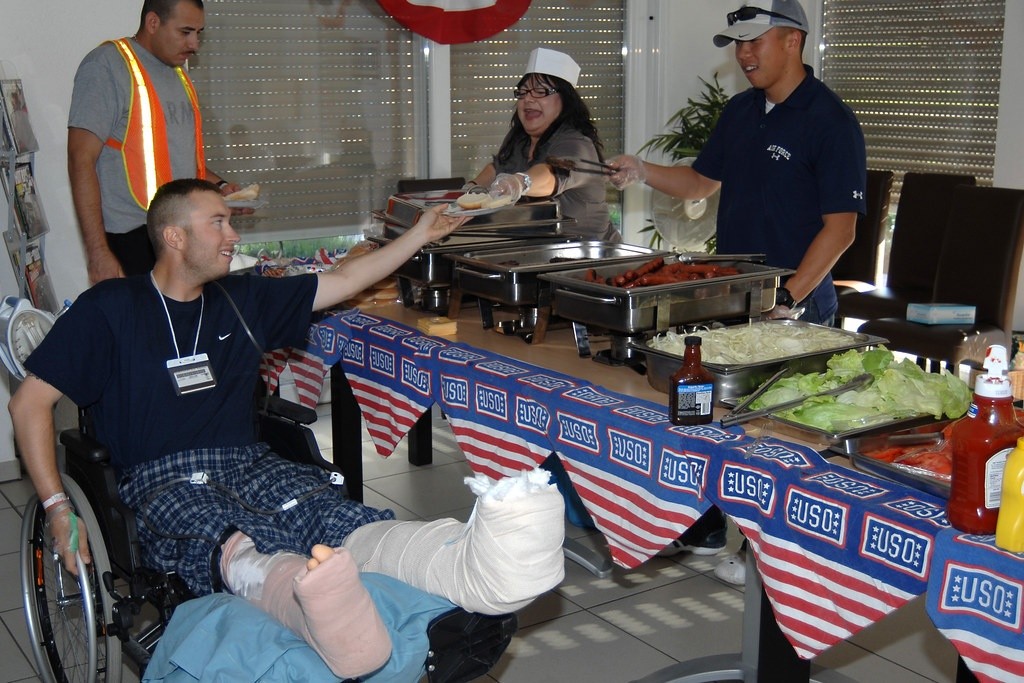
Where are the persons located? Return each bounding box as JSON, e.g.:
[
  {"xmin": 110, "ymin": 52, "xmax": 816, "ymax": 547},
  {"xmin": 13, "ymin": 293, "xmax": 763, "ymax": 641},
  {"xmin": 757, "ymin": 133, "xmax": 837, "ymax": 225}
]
[
  {"xmin": 461, "ymin": 49, "xmax": 628, "ymax": 245},
  {"xmin": 602, "ymin": 0, "xmax": 869, "ymax": 588},
  {"xmin": 67, "ymin": 0, "xmax": 253, "ymax": 287},
  {"xmin": 8, "ymin": 178, "xmax": 566, "ymax": 681}
]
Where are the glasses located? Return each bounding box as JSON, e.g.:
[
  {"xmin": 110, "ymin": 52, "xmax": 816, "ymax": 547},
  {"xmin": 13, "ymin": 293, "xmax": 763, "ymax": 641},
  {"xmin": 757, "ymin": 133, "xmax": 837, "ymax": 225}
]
[
  {"xmin": 727, "ymin": 7, "xmax": 803, "ymax": 26},
  {"xmin": 513, "ymin": 87, "xmax": 559, "ymax": 100}
]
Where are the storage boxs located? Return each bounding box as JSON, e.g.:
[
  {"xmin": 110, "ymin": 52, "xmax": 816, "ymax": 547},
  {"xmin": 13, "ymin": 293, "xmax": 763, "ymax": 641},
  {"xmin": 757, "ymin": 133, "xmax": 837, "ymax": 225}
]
[{"xmin": 906, "ymin": 303, "xmax": 976, "ymax": 325}]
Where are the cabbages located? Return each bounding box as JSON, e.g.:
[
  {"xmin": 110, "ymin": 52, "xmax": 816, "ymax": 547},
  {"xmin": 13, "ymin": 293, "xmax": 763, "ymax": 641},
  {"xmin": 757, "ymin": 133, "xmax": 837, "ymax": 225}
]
[{"xmin": 736, "ymin": 343, "xmax": 973, "ymax": 431}]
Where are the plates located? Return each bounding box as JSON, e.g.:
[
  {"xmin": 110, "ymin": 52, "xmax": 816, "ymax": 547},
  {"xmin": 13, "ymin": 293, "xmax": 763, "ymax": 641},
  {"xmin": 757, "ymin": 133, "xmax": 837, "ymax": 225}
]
[
  {"xmin": 440, "ymin": 198, "xmax": 515, "ymax": 219},
  {"xmin": 843, "ymin": 399, "xmax": 1024, "ymax": 454},
  {"xmin": 221, "ymin": 197, "xmax": 268, "ymax": 223}
]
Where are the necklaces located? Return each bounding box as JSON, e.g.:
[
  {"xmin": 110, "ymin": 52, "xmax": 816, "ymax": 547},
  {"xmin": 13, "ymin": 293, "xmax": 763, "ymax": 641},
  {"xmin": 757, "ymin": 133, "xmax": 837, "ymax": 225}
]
[{"xmin": 133, "ymin": 34, "xmax": 138, "ymax": 43}]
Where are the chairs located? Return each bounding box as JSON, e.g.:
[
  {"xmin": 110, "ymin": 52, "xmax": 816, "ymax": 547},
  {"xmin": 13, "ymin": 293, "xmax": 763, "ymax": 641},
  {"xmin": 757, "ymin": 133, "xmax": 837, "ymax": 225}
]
[{"xmin": 831, "ymin": 170, "xmax": 1024, "ymax": 389}]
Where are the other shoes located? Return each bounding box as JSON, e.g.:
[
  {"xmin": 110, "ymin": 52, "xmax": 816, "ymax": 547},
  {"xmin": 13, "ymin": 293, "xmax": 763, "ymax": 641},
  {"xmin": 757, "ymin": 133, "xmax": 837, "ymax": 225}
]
[
  {"xmin": 714, "ymin": 551, "xmax": 747, "ymax": 586},
  {"xmin": 654, "ymin": 540, "xmax": 726, "ymax": 557}
]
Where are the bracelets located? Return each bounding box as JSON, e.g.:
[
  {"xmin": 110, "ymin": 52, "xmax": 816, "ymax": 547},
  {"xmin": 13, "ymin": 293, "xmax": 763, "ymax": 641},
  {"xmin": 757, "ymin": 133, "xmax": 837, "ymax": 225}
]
[
  {"xmin": 215, "ymin": 180, "xmax": 228, "ymax": 189},
  {"xmin": 46, "ymin": 504, "xmax": 76, "ymax": 523},
  {"xmin": 467, "ymin": 180, "xmax": 478, "ymax": 185},
  {"xmin": 42, "ymin": 493, "xmax": 69, "ymax": 510}
]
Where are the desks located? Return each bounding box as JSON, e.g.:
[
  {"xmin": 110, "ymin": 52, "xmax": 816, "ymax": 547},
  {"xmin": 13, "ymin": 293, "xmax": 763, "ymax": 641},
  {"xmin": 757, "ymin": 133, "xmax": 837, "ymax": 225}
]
[{"xmin": 254, "ymin": 250, "xmax": 1024, "ymax": 683}]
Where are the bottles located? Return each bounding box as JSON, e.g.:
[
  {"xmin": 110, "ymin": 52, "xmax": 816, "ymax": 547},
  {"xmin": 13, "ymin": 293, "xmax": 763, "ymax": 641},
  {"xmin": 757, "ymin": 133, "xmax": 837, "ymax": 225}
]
[
  {"xmin": 669, "ymin": 336, "xmax": 714, "ymax": 426},
  {"xmin": 995, "ymin": 437, "xmax": 1024, "ymax": 552},
  {"xmin": 948, "ymin": 343, "xmax": 1024, "ymax": 534}
]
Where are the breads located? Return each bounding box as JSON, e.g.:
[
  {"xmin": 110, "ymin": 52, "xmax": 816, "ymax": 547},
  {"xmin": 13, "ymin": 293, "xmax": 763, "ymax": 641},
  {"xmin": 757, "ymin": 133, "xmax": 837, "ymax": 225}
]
[
  {"xmin": 332, "ymin": 241, "xmax": 401, "ymax": 303},
  {"xmin": 223, "ymin": 183, "xmax": 259, "ymax": 201},
  {"xmin": 455, "ymin": 192, "xmax": 511, "ymax": 209}
]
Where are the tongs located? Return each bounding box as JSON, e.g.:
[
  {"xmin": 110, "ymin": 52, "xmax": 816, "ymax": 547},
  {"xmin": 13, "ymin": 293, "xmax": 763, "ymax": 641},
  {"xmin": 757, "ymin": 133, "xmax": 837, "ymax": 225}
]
[
  {"xmin": 675, "ymin": 253, "xmax": 767, "ymax": 264},
  {"xmin": 721, "ymin": 366, "xmax": 875, "ymax": 428},
  {"xmin": 546, "ymin": 153, "xmax": 629, "ymax": 177}
]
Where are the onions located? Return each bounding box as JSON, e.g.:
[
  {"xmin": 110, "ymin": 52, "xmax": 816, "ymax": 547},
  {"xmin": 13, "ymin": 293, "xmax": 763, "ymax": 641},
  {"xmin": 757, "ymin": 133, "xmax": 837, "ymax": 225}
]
[{"xmin": 651, "ymin": 319, "xmax": 855, "ymax": 366}]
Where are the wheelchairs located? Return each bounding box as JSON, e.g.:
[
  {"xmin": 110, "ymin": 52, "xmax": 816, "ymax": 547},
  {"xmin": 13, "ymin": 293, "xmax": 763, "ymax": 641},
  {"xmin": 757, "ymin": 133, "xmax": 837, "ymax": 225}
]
[{"xmin": 20, "ymin": 295, "xmax": 516, "ymax": 683}]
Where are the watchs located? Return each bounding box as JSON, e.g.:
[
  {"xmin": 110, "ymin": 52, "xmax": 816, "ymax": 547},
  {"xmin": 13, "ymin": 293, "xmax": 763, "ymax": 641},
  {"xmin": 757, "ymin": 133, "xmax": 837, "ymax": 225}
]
[
  {"xmin": 516, "ymin": 171, "xmax": 531, "ymax": 196},
  {"xmin": 775, "ymin": 287, "xmax": 796, "ymax": 309}
]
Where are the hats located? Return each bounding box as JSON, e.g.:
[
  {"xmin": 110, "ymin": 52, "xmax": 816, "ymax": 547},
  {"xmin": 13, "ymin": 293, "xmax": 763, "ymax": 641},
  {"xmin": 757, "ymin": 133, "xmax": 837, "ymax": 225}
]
[
  {"xmin": 524, "ymin": 46, "xmax": 581, "ymax": 89},
  {"xmin": 713, "ymin": 0, "xmax": 809, "ymax": 48}
]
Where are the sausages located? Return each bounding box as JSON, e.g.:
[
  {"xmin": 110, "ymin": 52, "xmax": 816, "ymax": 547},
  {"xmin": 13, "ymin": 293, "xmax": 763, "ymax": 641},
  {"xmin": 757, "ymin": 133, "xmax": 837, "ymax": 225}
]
[{"xmin": 585, "ymin": 258, "xmax": 740, "ymax": 289}]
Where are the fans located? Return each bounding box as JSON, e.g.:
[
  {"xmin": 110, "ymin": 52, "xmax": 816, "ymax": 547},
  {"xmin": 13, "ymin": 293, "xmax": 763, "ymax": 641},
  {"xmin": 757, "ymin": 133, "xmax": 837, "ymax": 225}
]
[{"xmin": 651, "ymin": 157, "xmax": 721, "ymax": 251}]
